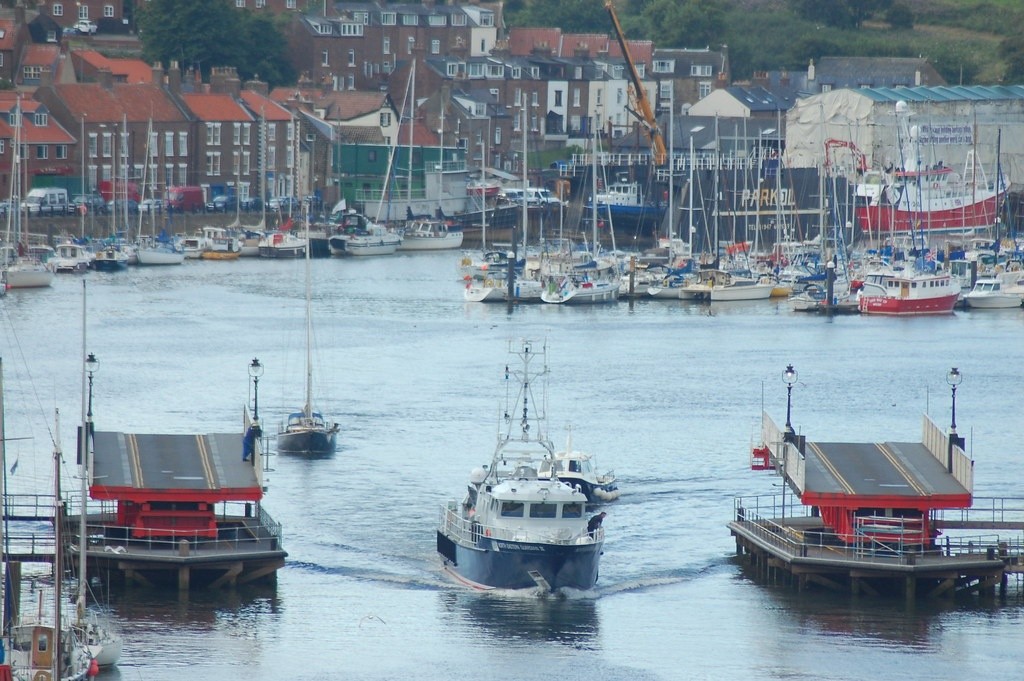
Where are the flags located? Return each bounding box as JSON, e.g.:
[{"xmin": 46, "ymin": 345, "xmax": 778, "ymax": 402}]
[
  {"xmin": 331, "ymin": 199, "xmax": 346, "ymax": 214},
  {"xmin": 922, "ymin": 247, "xmax": 938, "ymax": 271}
]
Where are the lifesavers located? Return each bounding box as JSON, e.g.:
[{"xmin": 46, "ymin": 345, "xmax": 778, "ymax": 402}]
[
  {"xmin": 1012, "ymin": 265, "xmax": 1019, "ymax": 271},
  {"xmin": 933, "ymin": 182, "xmax": 938, "ymax": 189},
  {"xmin": 485, "ymin": 529, "xmax": 490, "ymax": 537},
  {"xmin": 34, "ymin": 670, "xmax": 51, "ymax": 681},
  {"xmin": 304, "ymin": 418, "xmax": 313, "ymax": 428},
  {"xmin": 96, "ymin": 252, "xmax": 103, "ymax": 258},
  {"xmin": 484, "ymin": 277, "xmax": 494, "ymax": 287}
]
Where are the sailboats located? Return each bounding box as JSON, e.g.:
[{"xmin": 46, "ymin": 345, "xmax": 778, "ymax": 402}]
[
  {"xmin": 464, "ymin": 93, "xmax": 1024, "ymax": 320},
  {"xmin": 0, "ymin": 57, "xmax": 465, "ymax": 681}
]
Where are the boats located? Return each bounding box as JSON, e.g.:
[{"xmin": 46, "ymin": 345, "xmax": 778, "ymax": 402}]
[
  {"xmin": 434, "ymin": 334, "xmax": 608, "ymax": 598},
  {"xmin": 71, "ymin": 18, "xmax": 98, "ymax": 37},
  {"xmin": 274, "ymin": 200, "xmax": 339, "ymax": 461},
  {"xmin": 535, "ymin": 421, "xmax": 621, "ymax": 505}
]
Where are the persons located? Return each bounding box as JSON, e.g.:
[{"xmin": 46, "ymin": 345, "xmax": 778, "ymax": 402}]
[
  {"xmin": 587, "ymin": 511, "xmax": 607, "ymax": 538},
  {"xmin": 241, "ymin": 416, "xmax": 262, "ymax": 463}
]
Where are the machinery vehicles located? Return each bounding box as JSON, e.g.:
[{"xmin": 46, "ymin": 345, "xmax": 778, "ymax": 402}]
[{"xmin": 599, "ymin": 1, "xmax": 688, "ymax": 208}]
